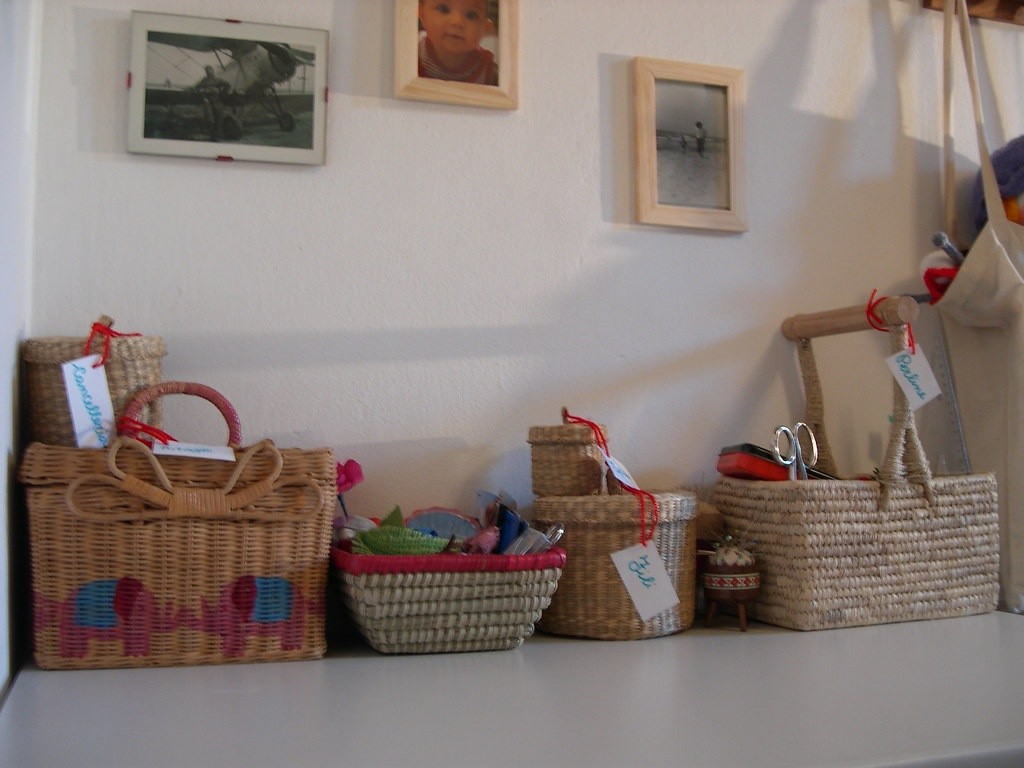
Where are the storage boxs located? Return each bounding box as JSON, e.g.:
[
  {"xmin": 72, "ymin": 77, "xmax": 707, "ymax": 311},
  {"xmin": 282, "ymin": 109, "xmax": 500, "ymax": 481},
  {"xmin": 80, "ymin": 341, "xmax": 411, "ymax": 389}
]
[{"xmin": 717, "ymin": 443, "xmax": 843, "ymax": 480}]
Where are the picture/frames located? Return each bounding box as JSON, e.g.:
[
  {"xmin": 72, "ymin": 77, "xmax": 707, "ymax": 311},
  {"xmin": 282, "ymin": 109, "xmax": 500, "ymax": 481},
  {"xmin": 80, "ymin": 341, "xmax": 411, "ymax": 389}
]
[
  {"xmin": 126, "ymin": 10, "xmax": 330, "ymax": 167},
  {"xmin": 394, "ymin": 0, "xmax": 520, "ymax": 110},
  {"xmin": 631, "ymin": 56, "xmax": 748, "ymax": 233}
]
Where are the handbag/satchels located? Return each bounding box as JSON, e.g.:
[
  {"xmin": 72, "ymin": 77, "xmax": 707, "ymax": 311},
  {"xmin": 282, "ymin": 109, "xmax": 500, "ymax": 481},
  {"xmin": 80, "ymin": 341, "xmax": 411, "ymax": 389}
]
[{"xmin": 929, "ymin": 208, "xmax": 1024, "ymax": 334}]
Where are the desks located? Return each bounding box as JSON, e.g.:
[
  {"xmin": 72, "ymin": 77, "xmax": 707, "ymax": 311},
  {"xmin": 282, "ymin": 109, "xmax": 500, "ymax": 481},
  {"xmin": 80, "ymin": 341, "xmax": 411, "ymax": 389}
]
[{"xmin": 0, "ymin": 609, "xmax": 1024, "ymax": 767}]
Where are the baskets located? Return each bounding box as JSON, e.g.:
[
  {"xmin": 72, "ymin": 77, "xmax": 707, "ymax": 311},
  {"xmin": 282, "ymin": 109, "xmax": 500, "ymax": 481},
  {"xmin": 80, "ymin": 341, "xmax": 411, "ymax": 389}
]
[
  {"xmin": 706, "ymin": 294, "xmax": 1001, "ymax": 630},
  {"xmin": 21, "ymin": 380, "xmax": 337, "ymax": 669},
  {"xmin": 326, "ymin": 538, "xmax": 567, "ymax": 655},
  {"xmin": 19, "ymin": 315, "xmax": 168, "ymax": 451},
  {"xmin": 532, "ymin": 458, "xmax": 697, "ymax": 641},
  {"xmin": 526, "ymin": 407, "xmax": 610, "ymax": 496}
]
